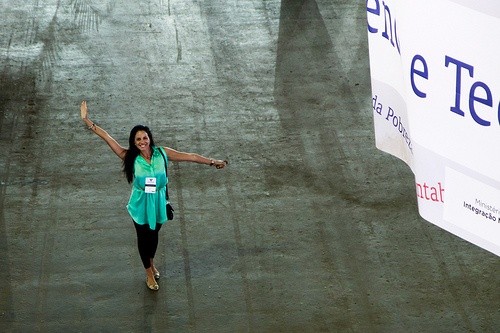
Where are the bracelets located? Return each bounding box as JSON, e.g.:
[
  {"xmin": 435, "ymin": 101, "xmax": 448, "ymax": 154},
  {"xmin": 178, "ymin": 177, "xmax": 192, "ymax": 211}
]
[
  {"xmin": 87, "ymin": 123, "xmax": 95, "ymax": 130},
  {"xmin": 209, "ymin": 158, "xmax": 214, "ymax": 166}
]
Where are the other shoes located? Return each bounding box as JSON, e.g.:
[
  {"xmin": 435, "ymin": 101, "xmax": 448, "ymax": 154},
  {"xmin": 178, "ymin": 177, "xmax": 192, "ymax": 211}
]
[
  {"xmin": 153, "ymin": 268, "xmax": 161, "ymax": 280},
  {"xmin": 146, "ymin": 276, "xmax": 159, "ymax": 291}
]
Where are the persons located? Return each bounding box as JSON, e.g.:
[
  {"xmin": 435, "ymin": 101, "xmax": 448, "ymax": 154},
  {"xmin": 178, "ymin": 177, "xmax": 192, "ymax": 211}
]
[{"xmin": 80, "ymin": 100, "xmax": 226, "ymax": 290}]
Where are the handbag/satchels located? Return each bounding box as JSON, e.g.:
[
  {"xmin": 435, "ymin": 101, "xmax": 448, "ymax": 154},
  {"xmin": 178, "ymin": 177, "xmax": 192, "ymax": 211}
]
[{"xmin": 165, "ymin": 197, "xmax": 175, "ymax": 221}]
